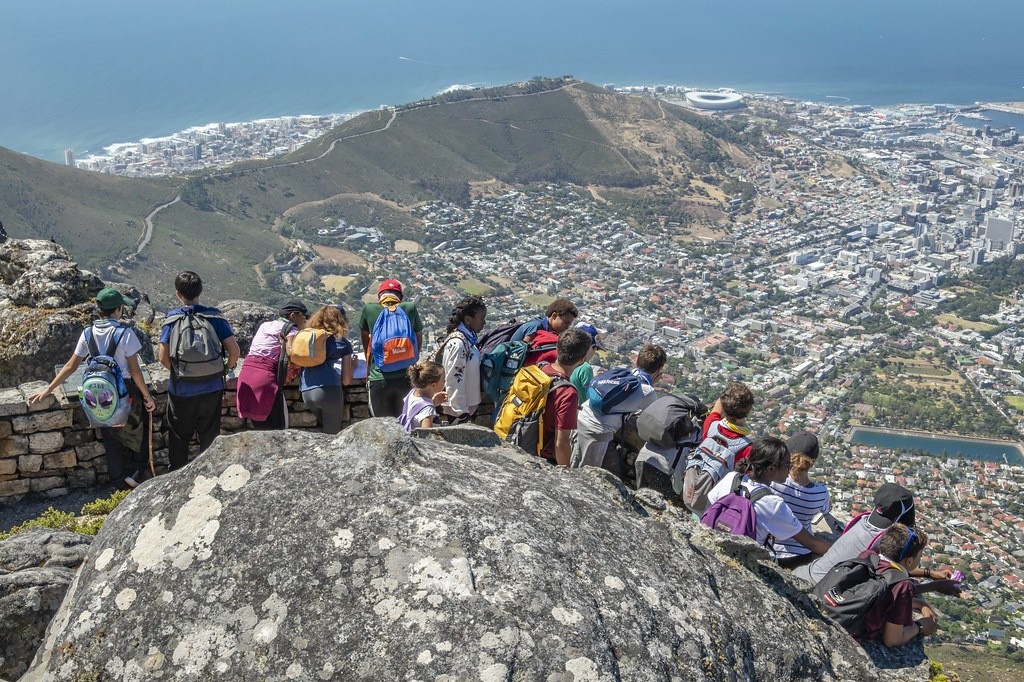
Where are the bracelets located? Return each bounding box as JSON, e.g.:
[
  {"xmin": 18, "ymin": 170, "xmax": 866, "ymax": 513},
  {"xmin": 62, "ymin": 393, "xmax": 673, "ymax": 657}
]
[
  {"xmin": 924, "ymin": 568, "xmax": 930, "ymax": 578},
  {"xmin": 915, "ymin": 621, "xmax": 924, "ymax": 634}
]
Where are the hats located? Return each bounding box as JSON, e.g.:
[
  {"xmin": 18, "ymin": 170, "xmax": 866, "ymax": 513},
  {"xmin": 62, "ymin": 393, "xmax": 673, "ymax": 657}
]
[
  {"xmin": 869, "ymin": 482, "xmax": 914, "ymax": 530},
  {"xmin": 324, "ymin": 302, "xmax": 349, "ymax": 323},
  {"xmin": 96, "ymin": 288, "xmax": 133, "ymax": 310},
  {"xmin": 788, "ymin": 431, "xmax": 819, "ymax": 460},
  {"xmin": 378, "ymin": 280, "xmax": 403, "ymax": 300},
  {"xmin": 280, "ymin": 299, "xmax": 310, "ymax": 320},
  {"xmin": 573, "ymin": 322, "xmax": 607, "ymax": 353}
]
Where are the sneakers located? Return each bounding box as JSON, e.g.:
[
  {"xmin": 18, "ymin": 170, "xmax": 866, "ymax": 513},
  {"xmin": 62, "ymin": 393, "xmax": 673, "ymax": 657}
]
[{"xmin": 125, "ymin": 470, "xmax": 143, "ymax": 486}]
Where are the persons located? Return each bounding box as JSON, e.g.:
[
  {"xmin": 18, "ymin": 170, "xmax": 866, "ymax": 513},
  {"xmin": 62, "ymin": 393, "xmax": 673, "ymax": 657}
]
[
  {"xmin": 480, "ymin": 321, "xmax": 590, "ymax": 424},
  {"xmin": 812, "ymin": 523, "xmax": 942, "ymax": 648},
  {"xmin": 291, "ymin": 303, "xmax": 357, "ymax": 436},
  {"xmin": 425, "ymin": 294, "xmax": 487, "ymax": 426},
  {"xmin": 698, "ymin": 436, "xmax": 833, "ymax": 558},
  {"xmin": 577, "ymin": 343, "xmax": 667, "ymax": 467},
  {"xmin": 791, "ymin": 482, "xmax": 965, "ymax": 599},
  {"xmin": 396, "ymin": 362, "xmax": 449, "ymax": 432},
  {"xmin": 682, "ymin": 381, "xmax": 753, "ymax": 524},
  {"xmin": 767, "ymin": 428, "xmax": 830, "ymax": 571},
  {"xmin": 158, "ymin": 271, "xmax": 240, "ymax": 473},
  {"xmin": 474, "ymin": 300, "xmax": 578, "ymax": 344},
  {"xmin": 633, "ymin": 393, "xmax": 710, "ymax": 506},
  {"xmin": 569, "ymin": 326, "xmax": 606, "ymax": 471},
  {"xmin": 27, "ymin": 285, "xmax": 155, "ymax": 491},
  {"xmin": 236, "ymin": 298, "xmax": 306, "ymax": 429},
  {"xmin": 494, "ymin": 327, "xmax": 591, "ymax": 470},
  {"xmin": 358, "ymin": 277, "xmax": 424, "ymax": 417}
]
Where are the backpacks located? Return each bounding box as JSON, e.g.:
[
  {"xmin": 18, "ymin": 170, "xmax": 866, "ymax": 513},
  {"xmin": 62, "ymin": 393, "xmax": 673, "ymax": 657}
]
[
  {"xmin": 163, "ymin": 307, "xmax": 234, "ymax": 380},
  {"xmin": 77, "ymin": 326, "xmax": 134, "ymax": 425},
  {"xmin": 478, "ymin": 317, "xmax": 546, "ymax": 353},
  {"xmin": 396, "ymin": 391, "xmax": 434, "ymax": 434},
  {"xmin": 636, "ymin": 392, "xmax": 710, "ymax": 448},
  {"xmin": 809, "ymin": 554, "xmax": 913, "ymax": 640},
  {"xmin": 681, "ymin": 417, "xmax": 753, "ymax": 516},
  {"xmin": 289, "ymin": 327, "xmax": 334, "ymax": 367},
  {"xmin": 480, "ymin": 333, "xmax": 562, "ymax": 403},
  {"xmin": 698, "ymin": 470, "xmax": 772, "ymax": 543},
  {"xmin": 369, "ymin": 305, "xmax": 419, "ymax": 373},
  {"xmin": 427, "ymin": 331, "xmax": 468, "ymax": 368},
  {"xmin": 590, "ymin": 368, "xmax": 651, "ymax": 415},
  {"xmin": 492, "ymin": 363, "xmax": 576, "ymax": 462}
]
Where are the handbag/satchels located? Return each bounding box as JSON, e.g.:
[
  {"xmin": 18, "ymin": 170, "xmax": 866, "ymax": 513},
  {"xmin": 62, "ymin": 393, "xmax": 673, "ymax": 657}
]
[{"xmin": 278, "ymin": 323, "xmax": 303, "ymax": 388}]
